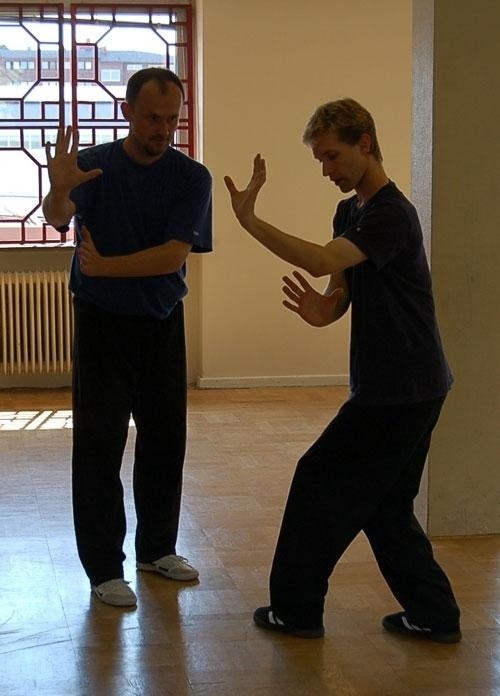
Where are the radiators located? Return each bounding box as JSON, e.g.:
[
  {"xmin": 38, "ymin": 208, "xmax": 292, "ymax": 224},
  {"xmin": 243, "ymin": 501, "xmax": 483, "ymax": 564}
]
[{"xmin": 0, "ymin": 270, "xmax": 73, "ymax": 375}]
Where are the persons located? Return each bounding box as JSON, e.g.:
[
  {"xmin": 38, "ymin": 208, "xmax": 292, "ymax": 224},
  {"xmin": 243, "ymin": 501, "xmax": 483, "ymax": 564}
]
[
  {"xmin": 39, "ymin": 66, "xmax": 215, "ymax": 611},
  {"xmin": 220, "ymin": 98, "xmax": 462, "ymax": 645}
]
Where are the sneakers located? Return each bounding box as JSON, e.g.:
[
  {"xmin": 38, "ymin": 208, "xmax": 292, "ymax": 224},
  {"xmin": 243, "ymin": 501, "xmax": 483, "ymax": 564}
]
[
  {"xmin": 134, "ymin": 552, "xmax": 200, "ymax": 582},
  {"xmin": 89, "ymin": 578, "xmax": 138, "ymax": 608},
  {"xmin": 251, "ymin": 605, "xmax": 326, "ymax": 640},
  {"xmin": 380, "ymin": 611, "xmax": 462, "ymax": 644}
]
